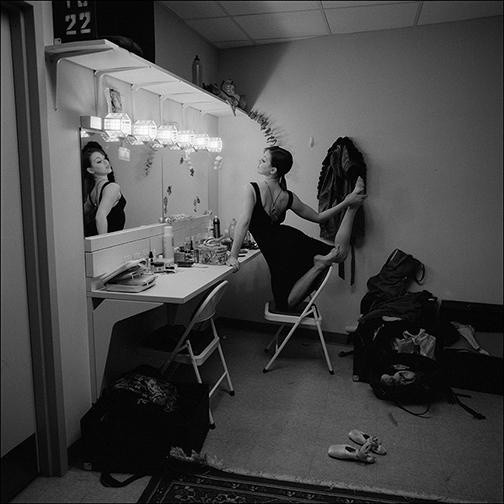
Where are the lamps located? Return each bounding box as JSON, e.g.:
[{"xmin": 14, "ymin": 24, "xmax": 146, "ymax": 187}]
[
  {"xmin": 178, "ymin": 129, "xmax": 194, "ymax": 151},
  {"xmin": 134, "ymin": 118, "xmax": 158, "ymax": 144},
  {"xmin": 158, "ymin": 123, "xmax": 178, "ymax": 146},
  {"xmin": 193, "ymin": 133, "xmax": 207, "ymax": 152},
  {"xmin": 206, "ymin": 136, "xmax": 223, "ymax": 154},
  {"xmin": 104, "ymin": 112, "xmax": 133, "ymax": 142}
]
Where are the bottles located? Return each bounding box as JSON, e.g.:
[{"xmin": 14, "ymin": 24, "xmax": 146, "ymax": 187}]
[{"xmin": 213, "ymin": 216, "xmax": 221, "ymax": 238}]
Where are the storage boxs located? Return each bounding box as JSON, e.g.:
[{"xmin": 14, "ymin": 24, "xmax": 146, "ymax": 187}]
[{"xmin": 53, "ymin": 0, "xmax": 158, "ymax": 63}]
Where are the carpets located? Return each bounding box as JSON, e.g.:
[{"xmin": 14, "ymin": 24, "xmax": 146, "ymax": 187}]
[{"xmin": 137, "ymin": 455, "xmax": 435, "ymax": 499}]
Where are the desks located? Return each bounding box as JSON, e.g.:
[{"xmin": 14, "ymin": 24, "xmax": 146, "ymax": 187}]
[{"xmin": 84, "ymin": 210, "xmax": 261, "ymax": 304}]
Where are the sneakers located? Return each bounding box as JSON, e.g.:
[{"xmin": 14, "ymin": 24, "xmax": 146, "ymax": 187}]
[
  {"xmin": 348, "ymin": 428, "xmax": 387, "ymax": 456},
  {"xmin": 328, "ymin": 444, "xmax": 377, "ymax": 465}
]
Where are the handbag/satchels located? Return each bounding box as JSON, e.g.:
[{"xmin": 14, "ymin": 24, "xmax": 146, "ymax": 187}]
[
  {"xmin": 368, "ymin": 344, "xmax": 445, "ymax": 418},
  {"xmin": 367, "ymin": 248, "xmax": 428, "ymax": 301}
]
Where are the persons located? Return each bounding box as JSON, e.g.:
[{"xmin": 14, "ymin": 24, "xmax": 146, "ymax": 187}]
[
  {"xmin": 80, "ymin": 146, "xmax": 127, "ymax": 237},
  {"xmin": 225, "ymin": 145, "xmax": 368, "ymax": 312}
]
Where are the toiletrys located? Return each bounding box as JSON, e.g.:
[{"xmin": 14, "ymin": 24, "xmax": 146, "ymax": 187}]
[
  {"xmin": 147, "ymin": 250, "xmax": 155, "ymax": 271},
  {"xmin": 154, "ymin": 261, "xmax": 165, "ymax": 272},
  {"xmin": 194, "ymin": 249, "xmax": 200, "ymax": 263}
]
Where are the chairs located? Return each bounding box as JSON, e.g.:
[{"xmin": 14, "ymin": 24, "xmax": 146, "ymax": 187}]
[
  {"xmin": 262, "ymin": 257, "xmax": 336, "ymax": 375},
  {"xmin": 141, "ymin": 280, "xmax": 241, "ymax": 430}
]
